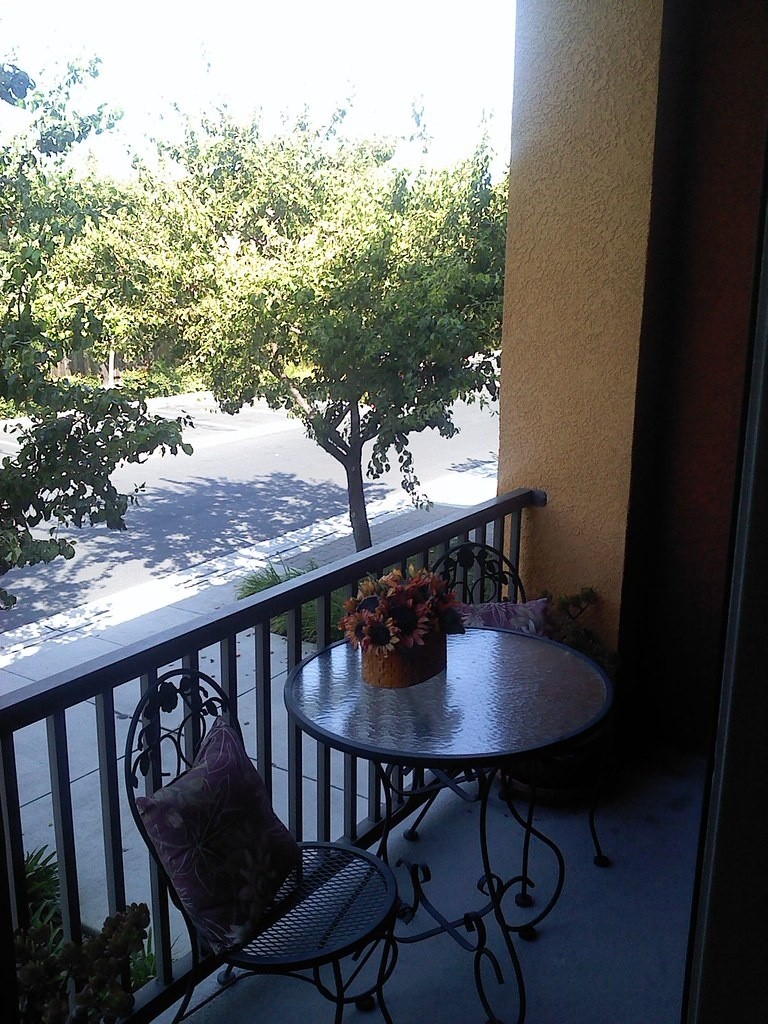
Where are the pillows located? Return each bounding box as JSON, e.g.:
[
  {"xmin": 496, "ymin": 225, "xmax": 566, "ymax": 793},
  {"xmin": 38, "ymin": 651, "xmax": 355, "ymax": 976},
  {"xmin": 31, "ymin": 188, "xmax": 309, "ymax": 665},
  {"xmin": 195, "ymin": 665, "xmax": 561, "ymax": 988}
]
[
  {"xmin": 456, "ymin": 591, "xmax": 553, "ymax": 639},
  {"xmin": 134, "ymin": 716, "xmax": 302, "ymax": 957}
]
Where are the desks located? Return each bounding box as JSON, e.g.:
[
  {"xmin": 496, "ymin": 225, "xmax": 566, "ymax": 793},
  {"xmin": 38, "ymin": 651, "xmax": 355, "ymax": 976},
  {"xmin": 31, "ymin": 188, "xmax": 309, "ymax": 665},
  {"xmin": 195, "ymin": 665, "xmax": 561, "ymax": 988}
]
[{"xmin": 285, "ymin": 626, "xmax": 613, "ymax": 1024}]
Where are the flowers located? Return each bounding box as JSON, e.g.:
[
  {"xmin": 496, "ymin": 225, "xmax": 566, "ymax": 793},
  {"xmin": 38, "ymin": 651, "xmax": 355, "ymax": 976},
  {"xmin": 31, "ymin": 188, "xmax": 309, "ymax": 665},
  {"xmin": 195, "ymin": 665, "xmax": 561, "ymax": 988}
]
[{"xmin": 337, "ymin": 563, "xmax": 465, "ymax": 658}]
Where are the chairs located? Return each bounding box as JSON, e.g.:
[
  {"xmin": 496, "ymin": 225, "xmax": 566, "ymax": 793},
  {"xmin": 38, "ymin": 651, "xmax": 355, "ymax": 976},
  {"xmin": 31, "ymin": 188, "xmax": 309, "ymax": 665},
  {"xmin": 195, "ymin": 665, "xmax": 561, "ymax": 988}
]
[
  {"xmin": 403, "ymin": 541, "xmax": 615, "ymax": 908},
  {"xmin": 125, "ymin": 669, "xmax": 409, "ymax": 1024}
]
